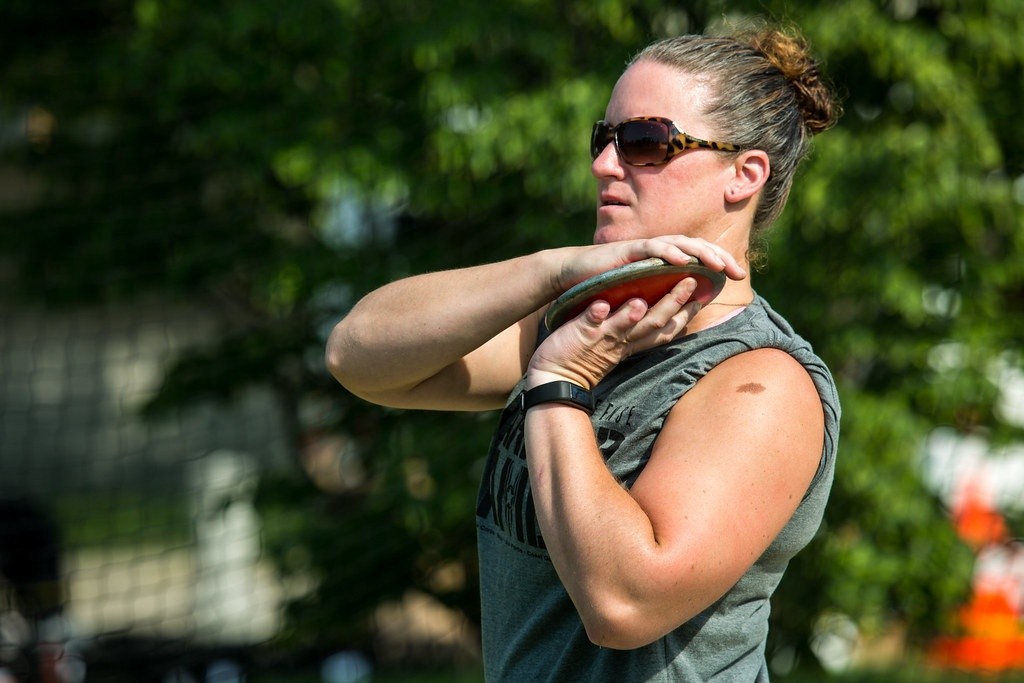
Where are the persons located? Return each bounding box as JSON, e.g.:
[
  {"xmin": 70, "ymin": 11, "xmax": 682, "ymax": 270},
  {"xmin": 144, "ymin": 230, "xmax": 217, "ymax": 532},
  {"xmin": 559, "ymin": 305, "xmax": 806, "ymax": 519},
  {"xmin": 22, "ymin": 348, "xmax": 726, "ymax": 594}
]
[{"xmin": 325, "ymin": 28, "xmax": 840, "ymax": 683}]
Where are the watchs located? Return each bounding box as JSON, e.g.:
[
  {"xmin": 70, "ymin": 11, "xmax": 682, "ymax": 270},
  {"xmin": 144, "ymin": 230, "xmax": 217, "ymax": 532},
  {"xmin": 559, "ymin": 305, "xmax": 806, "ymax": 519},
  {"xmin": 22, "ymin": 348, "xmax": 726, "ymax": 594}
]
[{"xmin": 520, "ymin": 381, "xmax": 595, "ymax": 415}]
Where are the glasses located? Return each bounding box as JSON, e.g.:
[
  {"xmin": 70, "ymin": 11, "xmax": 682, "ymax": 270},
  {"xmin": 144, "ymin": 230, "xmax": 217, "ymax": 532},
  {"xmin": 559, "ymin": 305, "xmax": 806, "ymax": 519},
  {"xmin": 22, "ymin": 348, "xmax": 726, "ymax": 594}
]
[{"xmin": 590, "ymin": 117, "xmax": 773, "ymax": 183}]
